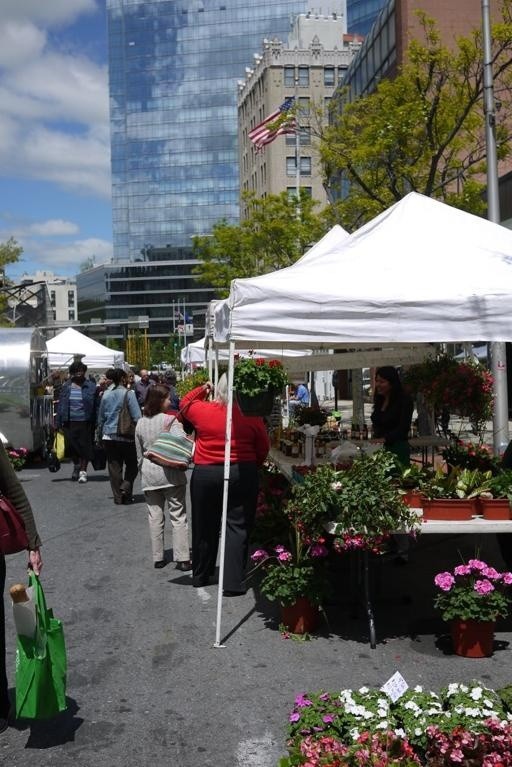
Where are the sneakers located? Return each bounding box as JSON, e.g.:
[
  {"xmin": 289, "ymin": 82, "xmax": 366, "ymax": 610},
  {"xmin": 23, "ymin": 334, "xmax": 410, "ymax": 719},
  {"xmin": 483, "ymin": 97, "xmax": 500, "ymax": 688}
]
[
  {"xmin": 152, "ymin": 555, "xmax": 251, "ymax": 599},
  {"xmin": 70, "ymin": 471, "xmax": 137, "ymax": 507}
]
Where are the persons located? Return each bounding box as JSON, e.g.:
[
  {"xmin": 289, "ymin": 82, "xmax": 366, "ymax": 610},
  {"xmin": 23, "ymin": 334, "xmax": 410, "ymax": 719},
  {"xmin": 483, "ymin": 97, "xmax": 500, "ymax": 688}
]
[
  {"xmin": 58, "ymin": 360, "xmax": 191, "ymax": 503},
  {"xmin": 133, "ymin": 383, "xmax": 192, "ymax": 572},
  {"xmin": 371, "ymin": 365, "xmax": 413, "ymax": 470},
  {"xmin": 0, "ymin": 441, "xmax": 44, "ymax": 730},
  {"xmin": 293, "ymin": 386, "xmax": 312, "ymax": 407},
  {"xmin": 178, "ymin": 371, "xmax": 269, "ymax": 597}
]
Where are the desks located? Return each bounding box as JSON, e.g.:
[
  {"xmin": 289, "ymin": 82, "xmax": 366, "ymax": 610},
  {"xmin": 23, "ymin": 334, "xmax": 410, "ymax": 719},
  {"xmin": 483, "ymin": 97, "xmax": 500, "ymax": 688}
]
[
  {"xmin": 265, "ymin": 445, "xmax": 333, "ymax": 469},
  {"xmin": 404, "ymin": 433, "xmax": 450, "ymax": 468},
  {"xmin": 277, "ymin": 462, "xmax": 512, "ymax": 649}
]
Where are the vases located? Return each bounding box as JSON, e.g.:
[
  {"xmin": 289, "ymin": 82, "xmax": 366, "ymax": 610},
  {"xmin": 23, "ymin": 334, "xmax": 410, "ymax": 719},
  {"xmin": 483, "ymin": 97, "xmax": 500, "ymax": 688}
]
[
  {"xmin": 235, "ymin": 386, "xmax": 276, "ymax": 418},
  {"xmin": 450, "ymin": 613, "xmax": 497, "ymax": 657},
  {"xmin": 277, "ymin": 595, "xmax": 318, "ymax": 635}
]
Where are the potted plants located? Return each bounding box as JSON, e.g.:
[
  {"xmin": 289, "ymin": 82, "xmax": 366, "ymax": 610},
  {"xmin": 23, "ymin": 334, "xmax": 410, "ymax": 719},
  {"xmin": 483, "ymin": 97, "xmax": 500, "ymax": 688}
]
[
  {"xmin": 393, "ymin": 460, "xmax": 434, "ymax": 508},
  {"xmin": 415, "ymin": 462, "xmax": 491, "ymax": 520},
  {"xmin": 478, "ymin": 477, "xmax": 512, "ymax": 520},
  {"xmin": 464, "ymin": 469, "xmax": 491, "ymax": 514}
]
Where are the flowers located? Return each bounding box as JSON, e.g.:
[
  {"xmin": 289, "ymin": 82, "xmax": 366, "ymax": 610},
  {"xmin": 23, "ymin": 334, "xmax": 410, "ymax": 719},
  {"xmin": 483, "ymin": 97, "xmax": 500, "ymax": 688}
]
[
  {"xmin": 432, "ymin": 556, "xmax": 512, "ymax": 622},
  {"xmin": 279, "ymin": 459, "xmax": 341, "ymax": 559},
  {"xmin": 231, "ymin": 351, "xmax": 290, "ymax": 398},
  {"xmin": 242, "ymin": 545, "xmax": 318, "ymax": 608},
  {"xmin": 332, "ymin": 444, "xmax": 428, "ymax": 557}
]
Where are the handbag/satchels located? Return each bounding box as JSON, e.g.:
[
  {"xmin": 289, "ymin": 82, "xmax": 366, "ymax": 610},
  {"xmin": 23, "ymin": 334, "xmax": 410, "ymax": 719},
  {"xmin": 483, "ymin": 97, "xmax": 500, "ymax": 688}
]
[
  {"xmin": 92, "ymin": 441, "xmax": 105, "ymax": 474},
  {"xmin": 54, "ymin": 430, "xmax": 65, "ymax": 460},
  {"xmin": 13, "ymin": 566, "xmax": 69, "ymax": 721},
  {"xmin": 45, "ymin": 450, "xmax": 61, "ymax": 475},
  {"xmin": 145, "ymin": 430, "xmax": 194, "ymax": 472},
  {"xmin": 117, "ymin": 404, "xmax": 140, "ymax": 440},
  {"xmin": 0, "ymin": 493, "xmax": 31, "ymax": 556}
]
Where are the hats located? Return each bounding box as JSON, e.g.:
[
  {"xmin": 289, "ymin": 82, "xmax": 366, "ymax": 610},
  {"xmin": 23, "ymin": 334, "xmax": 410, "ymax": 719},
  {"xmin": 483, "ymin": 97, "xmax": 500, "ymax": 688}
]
[{"xmin": 68, "ymin": 361, "xmax": 87, "ymax": 373}]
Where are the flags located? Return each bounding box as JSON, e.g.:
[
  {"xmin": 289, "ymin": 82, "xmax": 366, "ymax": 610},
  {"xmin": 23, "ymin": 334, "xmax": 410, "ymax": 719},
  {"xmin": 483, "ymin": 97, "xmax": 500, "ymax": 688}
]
[{"xmin": 248, "ymin": 99, "xmax": 297, "ymax": 148}]
